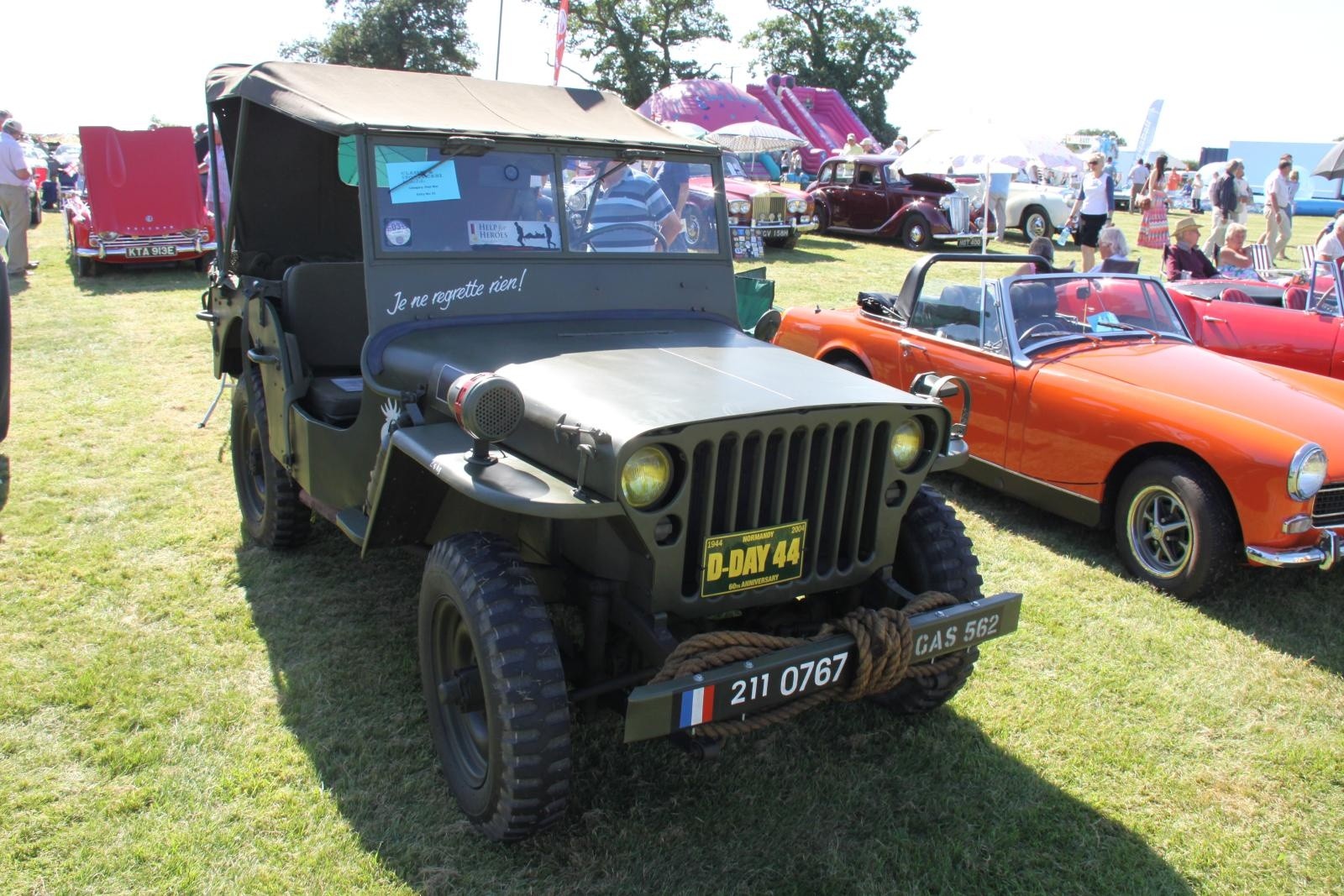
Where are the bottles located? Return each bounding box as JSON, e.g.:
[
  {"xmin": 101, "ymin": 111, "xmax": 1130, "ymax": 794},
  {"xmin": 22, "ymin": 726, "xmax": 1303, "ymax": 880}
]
[{"xmin": 1058, "ymin": 226, "xmax": 1071, "ymax": 246}]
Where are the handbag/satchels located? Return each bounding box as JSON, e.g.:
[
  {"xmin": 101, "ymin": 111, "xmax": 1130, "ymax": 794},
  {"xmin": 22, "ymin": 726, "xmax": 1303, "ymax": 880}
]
[{"xmin": 1135, "ymin": 193, "xmax": 1150, "ymax": 211}]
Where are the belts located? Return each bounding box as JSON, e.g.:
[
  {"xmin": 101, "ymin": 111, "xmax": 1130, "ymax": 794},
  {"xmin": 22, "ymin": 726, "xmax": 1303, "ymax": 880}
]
[{"xmin": 1266, "ymin": 204, "xmax": 1283, "ymax": 209}]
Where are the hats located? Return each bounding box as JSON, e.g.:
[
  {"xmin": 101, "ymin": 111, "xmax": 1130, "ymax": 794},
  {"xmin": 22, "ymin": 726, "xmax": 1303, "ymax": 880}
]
[
  {"xmin": 860, "ymin": 138, "xmax": 872, "ymax": 145},
  {"xmin": 847, "ymin": 133, "xmax": 855, "ymax": 141},
  {"xmin": 4, "ymin": 119, "xmax": 22, "ymax": 133},
  {"xmin": 893, "ymin": 140, "xmax": 907, "ymax": 149},
  {"xmin": 1168, "ymin": 217, "xmax": 1203, "ymax": 237},
  {"xmin": 203, "ymin": 123, "xmax": 219, "ymax": 134}
]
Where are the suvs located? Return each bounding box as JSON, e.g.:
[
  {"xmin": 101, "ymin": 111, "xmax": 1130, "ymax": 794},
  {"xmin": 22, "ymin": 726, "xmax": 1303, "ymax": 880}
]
[{"xmin": 196, "ymin": 57, "xmax": 1022, "ymax": 845}]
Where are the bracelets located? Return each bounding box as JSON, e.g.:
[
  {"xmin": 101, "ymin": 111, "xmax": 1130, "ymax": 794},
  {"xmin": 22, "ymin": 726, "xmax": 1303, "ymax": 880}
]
[
  {"xmin": 1107, "ymin": 218, "xmax": 1112, "ymax": 220},
  {"xmin": 1069, "ymin": 215, "xmax": 1073, "ymax": 219}
]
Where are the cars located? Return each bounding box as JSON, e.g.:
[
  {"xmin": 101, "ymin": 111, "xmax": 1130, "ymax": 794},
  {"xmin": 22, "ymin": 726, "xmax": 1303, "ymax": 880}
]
[
  {"xmin": 64, "ymin": 126, "xmax": 218, "ymax": 277},
  {"xmin": 564, "ymin": 147, "xmax": 1079, "ymax": 249}
]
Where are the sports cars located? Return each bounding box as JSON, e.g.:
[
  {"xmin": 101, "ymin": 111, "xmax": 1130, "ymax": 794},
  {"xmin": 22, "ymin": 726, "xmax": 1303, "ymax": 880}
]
[{"xmin": 769, "ymin": 250, "xmax": 1343, "ymax": 601}]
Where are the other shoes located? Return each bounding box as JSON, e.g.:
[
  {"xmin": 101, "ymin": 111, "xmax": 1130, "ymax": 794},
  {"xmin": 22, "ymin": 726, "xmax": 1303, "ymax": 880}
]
[
  {"xmin": 1272, "ymin": 262, "xmax": 1276, "ymax": 267},
  {"xmin": 1277, "ymin": 255, "xmax": 1289, "ymax": 260},
  {"xmin": 8, "ymin": 270, "xmax": 33, "ymax": 276},
  {"xmin": 27, "ymin": 259, "xmax": 39, "ymax": 269}
]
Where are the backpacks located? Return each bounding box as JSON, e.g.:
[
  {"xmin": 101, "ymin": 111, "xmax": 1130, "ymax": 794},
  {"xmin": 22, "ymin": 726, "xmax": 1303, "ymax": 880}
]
[{"xmin": 1210, "ymin": 177, "xmax": 1224, "ymax": 206}]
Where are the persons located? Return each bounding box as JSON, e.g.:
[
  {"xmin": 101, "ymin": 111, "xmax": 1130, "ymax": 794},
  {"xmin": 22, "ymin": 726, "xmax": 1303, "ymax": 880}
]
[
  {"xmin": 840, "ymin": 133, "xmax": 909, "ymax": 176},
  {"xmin": 1202, "ymin": 153, "xmax": 1300, "ymax": 268},
  {"xmin": 0, "ymin": 108, "xmax": 40, "ymax": 276},
  {"xmin": 1086, "ymin": 227, "xmax": 1129, "ymax": 274},
  {"xmin": 983, "ymin": 172, "xmax": 1017, "ymax": 242},
  {"xmin": 1102, "ymin": 157, "xmax": 1222, "ymax": 280},
  {"xmin": 585, "ymin": 160, "xmax": 683, "ymax": 252},
  {"xmin": 537, "ymin": 175, "xmax": 578, "ymax": 243},
  {"xmin": 1065, "ymin": 153, "xmax": 1114, "ymax": 273},
  {"xmin": 781, "ymin": 150, "xmax": 802, "ymax": 175},
  {"xmin": 647, "ymin": 160, "xmax": 690, "ymax": 252},
  {"xmin": 1314, "ymin": 209, "xmax": 1344, "ymax": 272},
  {"xmin": 194, "ymin": 122, "xmax": 230, "ymax": 224},
  {"xmin": 1018, "ymin": 163, "xmax": 1082, "ymax": 189},
  {"xmin": 1012, "ymin": 236, "xmax": 1054, "ymax": 283}
]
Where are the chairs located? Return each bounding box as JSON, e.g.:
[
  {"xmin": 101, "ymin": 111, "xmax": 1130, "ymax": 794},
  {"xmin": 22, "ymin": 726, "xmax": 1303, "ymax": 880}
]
[
  {"xmin": 1160, "ymin": 242, "xmax": 1170, "ymax": 280},
  {"xmin": 1211, "ymin": 242, "xmax": 1222, "ymax": 269},
  {"xmin": 939, "ymin": 286, "xmax": 999, "ymax": 345},
  {"xmin": 1220, "ymin": 288, "xmax": 1254, "ymax": 304},
  {"xmin": 859, "ymin": 171, "xmax": 872, "ymax": 185},
  {"xmin": 1010, "ymin": 282, "xmax": 1069, "ymax": 349},
  {"xmin": 282, "ymin": 262, "xmax": 368, "ymax": 420},
  {"xmin": 1282, "ymin": 287, "xmax": 1316, "ymax": 310},
  {"xmin": 1035, "ymin": 260, "xmax": 1075, "ymax": 274},
  {"xmin": 1251, "ymin": 244, "xmax": 1273, "ymax": 271},
  {"xmin": 1299, "ymin": 245, "xmax": 1315, "ymax": 269},
  {"xmin": 1100, "ymin": 258, "xmax": 1141, "ymax": 274}
]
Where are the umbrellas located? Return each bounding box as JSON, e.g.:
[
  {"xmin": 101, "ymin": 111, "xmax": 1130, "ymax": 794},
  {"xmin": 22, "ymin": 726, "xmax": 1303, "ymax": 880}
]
[
  {"xmin": 892, "ymin": 124, "xmax": 1086, "ymax": 311},
  {"xmin": 703, "ymin": 120, "xmax": 809, "ymax": 171},
  {"xmin": 1308, "ymin": 139, "xmax": 1344, "ymax": 180}
]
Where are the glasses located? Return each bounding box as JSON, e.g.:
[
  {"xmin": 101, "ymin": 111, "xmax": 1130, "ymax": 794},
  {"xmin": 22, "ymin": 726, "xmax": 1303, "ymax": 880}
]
[{"xmin": 1089, "ymin": 161, "xmax": 1098, "ymax": 166}]
